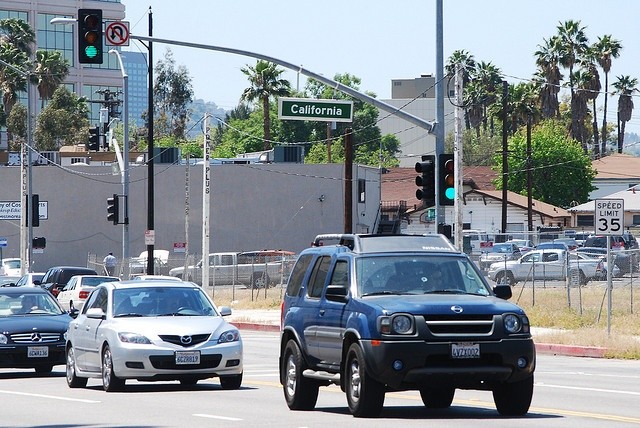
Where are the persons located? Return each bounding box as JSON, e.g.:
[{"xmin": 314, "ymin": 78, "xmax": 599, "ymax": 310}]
[
  {"xmin": 166, "ymin": 296, "xmax": 183, "ymax": 312},
  {"xmin": 16, "ymin": 295, "xmax": 46, "ymax": 314},
  {"xmin": 103, "ymin": 252, "xmax": 117, "ymax": 276},
  {"xmin": 426, "ymin": 270, "xmax": 459, "ymax": 289}
]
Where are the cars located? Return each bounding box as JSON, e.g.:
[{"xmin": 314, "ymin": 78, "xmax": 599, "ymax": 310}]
[
  {"xmin": 567, "ymin": 251, "xmax": 621, "ymax": 280},
  {"xmin": 552, "ymin": 237, "xmax": 580, "ymax": 251},
  {"xmin": 534, "ymin": 241, "xmax": 568, "ymax": 249},
  {"xmin": 0, "ymin": 258, "xmax": 29, "ymax": 278},
  {"xmin": 0, "ymin": 286, "xmax": 74, "ymax": 373},
  {"xmin": 573, "ymin": 245, "xmax": 638, "ymax": 278},
  {"xmin": 56, "ymin": 274, "xmax": 121, "ymax": 315},
  {"xmin": 65, "ymin": 279, "xmax": 243, "ymax": 391},
  {"xmin": 506, "ymin": 239, "xmax": 537, "ymax": 256},
  {"xmin": 479, "ymin": 243, "xmax": 522, "ymax": 270}
]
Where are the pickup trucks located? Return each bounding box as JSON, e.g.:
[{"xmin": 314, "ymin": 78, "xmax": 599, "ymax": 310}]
[
  {"xmin": 488, "ymin": 248, "xmax": 604, "ymax": 286},
  {"xmin": 169, "ymin": 250, "xmax": 283, "ymax": 288}
]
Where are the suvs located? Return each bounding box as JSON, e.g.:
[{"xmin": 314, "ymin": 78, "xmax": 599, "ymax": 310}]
[
  {"xmin": 279, "ymin": 234, "xmax": 536, "ymax": 415},
  {"xmin": 583, "ymin": 234, "xmax": 629, "ymax": 251}
]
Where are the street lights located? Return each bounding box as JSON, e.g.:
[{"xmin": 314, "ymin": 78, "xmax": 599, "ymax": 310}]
[
  {"xmin": 50, "ymin": 4, "xmax": 154, "ymax": 275},
  {"xmin": 109, "ymin": 48, "xmax": 129, "ymax": 279}
]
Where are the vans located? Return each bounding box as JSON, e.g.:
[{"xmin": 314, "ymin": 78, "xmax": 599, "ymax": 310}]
[
  {"xmin": 33, "ymin": 266, "xmax": 99, "ymax": 298},
  {"xmin": 458, "ymin": 229, "xmax": 493, "ymax": 253}
]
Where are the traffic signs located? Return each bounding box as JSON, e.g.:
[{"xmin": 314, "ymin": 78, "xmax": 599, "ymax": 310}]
[
  {"xmin": 594, "ymin": 198, "xmax": 624, "ymax": 235},
  {"xmin": 277, "ymin": 95, "xmax": 355, "ymax": 123}
]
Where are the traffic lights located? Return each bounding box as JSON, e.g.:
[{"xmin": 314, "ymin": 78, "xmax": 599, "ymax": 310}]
[
  {"xmin": 107, "ymin": 192, "xmax": 119, "ymax": 225},
  {"xmin": 415, "ymin": 154, "xmax": 435, "ymax": 205},
  {"xmin": 78, "ymin": 8, "xmax": 102, "ymax": 63},
  {"xmin": 437, "ymin": 152, "xmax": 454, "ymax": 206},
  {"xmin": 88, "ymin": 124, "xmax": 99, "ymax": 151}
]
